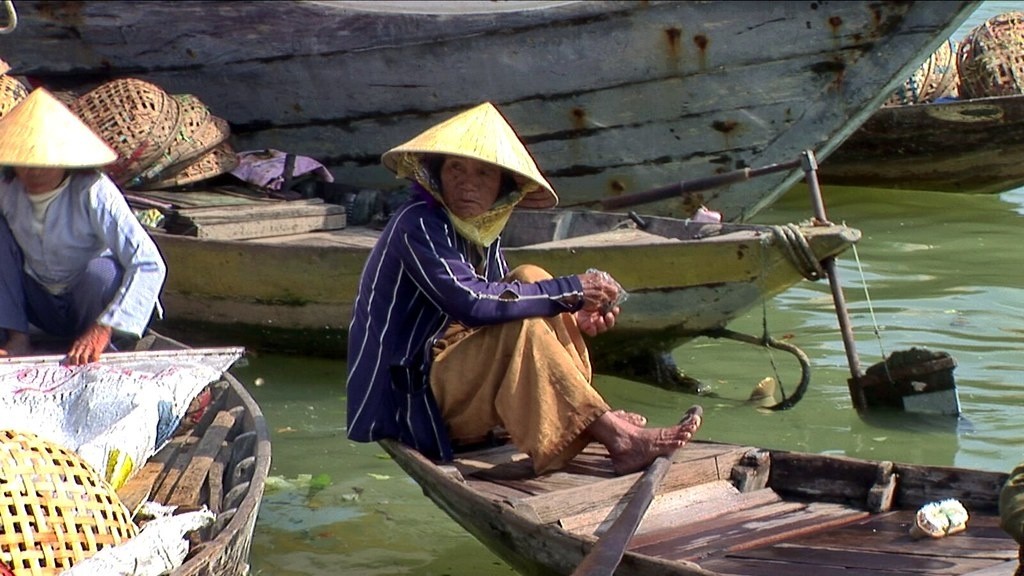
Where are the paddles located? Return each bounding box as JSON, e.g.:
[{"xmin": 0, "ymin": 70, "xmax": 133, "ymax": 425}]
[{"xmin": 568, "ymin": 404, "xmax": 704, "ymax": 576}]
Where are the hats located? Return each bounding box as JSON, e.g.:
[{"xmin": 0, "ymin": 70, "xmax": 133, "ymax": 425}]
[
  {"xmin": 381, "ymin": 101, "xmax": 558, "ymax": 211},
  {"xmin": 1, "ymin": 87, "xmax": 119, "ymax": 168}
]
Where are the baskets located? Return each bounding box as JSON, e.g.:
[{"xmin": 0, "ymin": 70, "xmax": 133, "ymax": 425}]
[
  {"xmin": 1, "ymin": 429, "xmax": 142, "ymax": 576},
  {"xmin": 880, "ymin": 9, "xmax": 1024, "ymax": 108},
  {"xmin": 0, "ymin": 61, "xmax": 241, "ymax": 191}
]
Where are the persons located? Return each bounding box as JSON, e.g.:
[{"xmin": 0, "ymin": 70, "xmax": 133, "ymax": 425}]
[
  {"xmin": 345, "ymin": 100, "xmax": 704, "ymax": 476},
  {"xmin": 0, "ymin": 88, "xmax": 167, "ymax": 367}
]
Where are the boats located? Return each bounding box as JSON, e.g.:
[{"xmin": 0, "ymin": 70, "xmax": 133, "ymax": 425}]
[
  {"xmin": 0, "ymin": 3, "xmax": 1022, "ymax": 368},
  {"xmin": 376, "ymin": 436, "xmax": 1020, "ymax": 576},
  {"xmin": 0, "ymin": 325, "xmax": 272, "ymax": 574}
]
